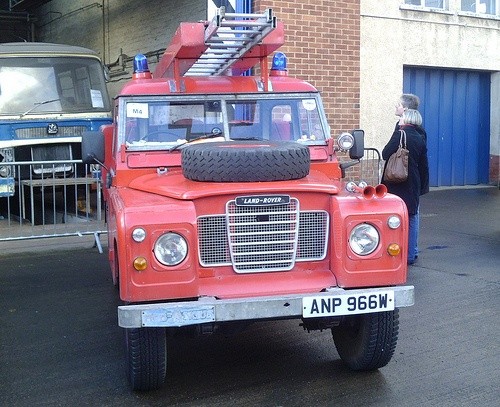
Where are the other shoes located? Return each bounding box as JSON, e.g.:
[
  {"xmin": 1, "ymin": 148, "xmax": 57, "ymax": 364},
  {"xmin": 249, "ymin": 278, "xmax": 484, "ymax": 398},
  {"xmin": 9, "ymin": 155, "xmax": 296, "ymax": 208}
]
[{"xmin": 407, "ymin": 256, "xmax": 417, "ymax": 264}]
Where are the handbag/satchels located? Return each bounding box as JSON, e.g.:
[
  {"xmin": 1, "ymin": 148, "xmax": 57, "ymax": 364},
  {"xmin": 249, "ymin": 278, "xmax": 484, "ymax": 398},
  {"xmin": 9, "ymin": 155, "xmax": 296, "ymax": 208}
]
[{"xmin": 383, "ymin": 130, "xmax": 410, "ymax": 183}]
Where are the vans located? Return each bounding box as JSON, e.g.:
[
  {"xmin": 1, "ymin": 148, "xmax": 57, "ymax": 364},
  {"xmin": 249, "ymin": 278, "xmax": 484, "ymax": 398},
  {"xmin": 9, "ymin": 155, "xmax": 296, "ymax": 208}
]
[{"xmin": 0, "ymin": 41, "xmax": 116, "ymax": 199}]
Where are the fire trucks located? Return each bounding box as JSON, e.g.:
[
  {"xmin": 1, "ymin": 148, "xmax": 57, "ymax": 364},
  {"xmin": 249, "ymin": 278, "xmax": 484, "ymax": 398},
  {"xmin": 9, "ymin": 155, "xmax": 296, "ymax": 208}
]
[{"xmin": 81, "ymin": 6, "xmax": 414, "ymax": 392}]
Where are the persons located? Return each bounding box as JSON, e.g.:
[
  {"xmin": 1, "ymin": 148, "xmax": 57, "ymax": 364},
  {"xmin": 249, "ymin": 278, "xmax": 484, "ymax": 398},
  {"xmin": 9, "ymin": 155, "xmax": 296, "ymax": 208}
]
[
  {"xmin": 394, "ymin": 94, "xmax": 427, "ymax": 141},
  {"xmin": 380, "ymin": 109, "xmax": 429, "ymax": 266}
]
